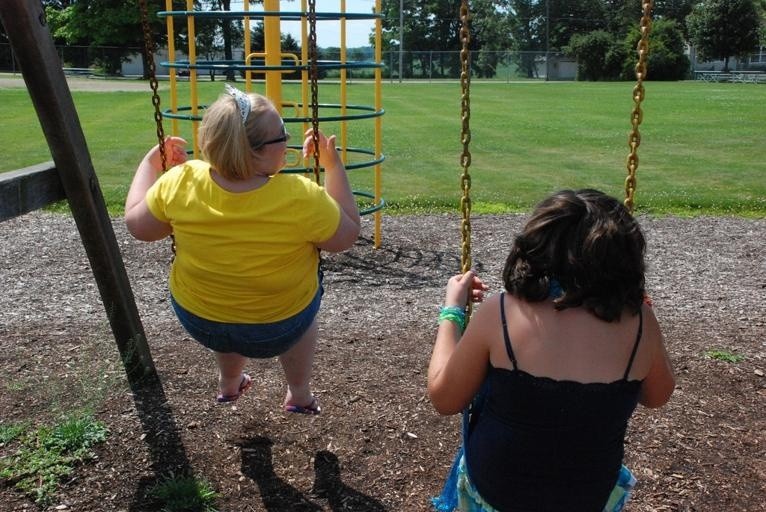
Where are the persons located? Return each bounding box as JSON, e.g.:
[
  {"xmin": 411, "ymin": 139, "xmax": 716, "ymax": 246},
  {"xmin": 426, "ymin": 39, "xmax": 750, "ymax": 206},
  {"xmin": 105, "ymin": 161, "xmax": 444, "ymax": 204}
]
[
  {"xmin": 426, "ymin": 186, "xmax": 677, "ymax": 512},
  {"xmin": 123, "ymin": 83, "xmax": 363, "ymax": 417}
]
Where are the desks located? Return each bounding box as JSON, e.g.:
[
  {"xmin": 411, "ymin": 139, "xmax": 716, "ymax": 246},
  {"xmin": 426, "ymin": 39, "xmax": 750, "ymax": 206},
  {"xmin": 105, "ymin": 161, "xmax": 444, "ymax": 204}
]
[
  {"xmin": 729, "ymin": 69, "xmax": 764, "ymax": 84},
  {"xmin": 694, "ymin": 68, "xmax": 722, "ymax": 84}
]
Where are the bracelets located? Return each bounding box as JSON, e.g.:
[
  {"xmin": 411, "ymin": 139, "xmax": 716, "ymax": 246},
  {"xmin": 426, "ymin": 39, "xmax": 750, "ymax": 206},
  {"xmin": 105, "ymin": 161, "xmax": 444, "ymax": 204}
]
[
  {"xmin": 436, "ymin": 305, "xmax": 466, "ymax": 333},
  {"xmin": 643, "ymin": 294, "xmax": 653, "ymax": 308}
]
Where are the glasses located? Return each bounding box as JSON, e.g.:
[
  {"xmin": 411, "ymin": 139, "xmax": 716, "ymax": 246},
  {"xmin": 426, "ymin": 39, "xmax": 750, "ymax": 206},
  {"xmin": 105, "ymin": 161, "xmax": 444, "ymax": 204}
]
[{"xmin": 263, "ymin": 128, "xmax": 291, "ymax": 145}]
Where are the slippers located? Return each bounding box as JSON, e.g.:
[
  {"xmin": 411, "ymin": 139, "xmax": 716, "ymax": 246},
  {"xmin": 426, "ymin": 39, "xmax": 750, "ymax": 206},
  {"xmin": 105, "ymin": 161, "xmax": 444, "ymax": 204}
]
[
  {"xmin": 285, "ymin": 396, "xmax": 321, "ymax": 414},
  {"xmin": 217, "ymin": 374, "xmax": 251, "ymax": 402}
]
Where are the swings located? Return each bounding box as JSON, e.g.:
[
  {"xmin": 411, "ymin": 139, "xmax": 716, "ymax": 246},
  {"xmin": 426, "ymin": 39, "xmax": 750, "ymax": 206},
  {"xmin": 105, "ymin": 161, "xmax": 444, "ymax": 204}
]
[
  {"xmin": 457, "ymin": 0, "xmax": 654, "ymax": 512},
  {"xmin": 138, "ymin": 1, "xmax": 324, "ymax": 298}
]
